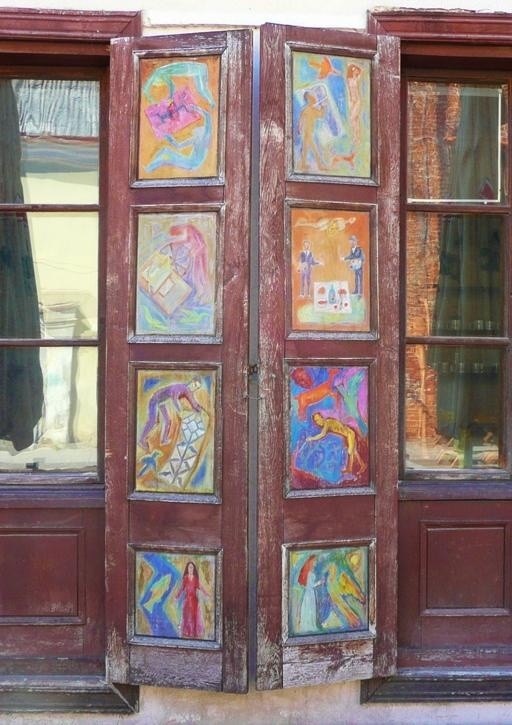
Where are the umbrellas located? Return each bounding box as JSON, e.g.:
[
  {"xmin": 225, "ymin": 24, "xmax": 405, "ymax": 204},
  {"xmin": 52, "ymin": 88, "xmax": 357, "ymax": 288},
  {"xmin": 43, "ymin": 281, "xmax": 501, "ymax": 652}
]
[
  {"xmin": 0, "ymin": 76, "xmax": 47, "ymax": 456},
  {"xmin": 424, "ymin": 82, "xmax": 509, "ymax": 468}
]
